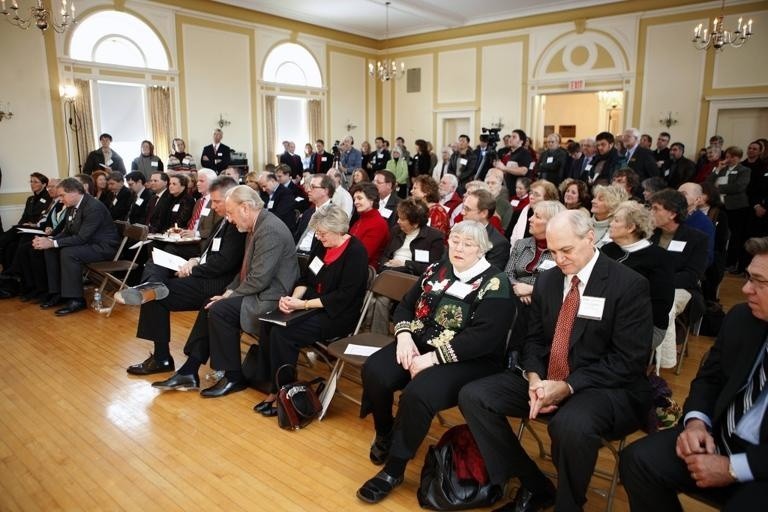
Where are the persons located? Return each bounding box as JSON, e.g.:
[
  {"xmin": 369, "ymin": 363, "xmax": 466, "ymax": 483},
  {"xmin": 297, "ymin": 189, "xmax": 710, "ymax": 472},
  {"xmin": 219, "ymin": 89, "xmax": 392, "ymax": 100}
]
[
  {"xmin": 355, "ymin": 220, "xmax": 513, "ymax": 504},
  {"xmin": 619, "ymin": 237, "xmax": 768, "ymax": 512},
  {"xmin": 0, "ymin": 128, "xmax": 768, "ymax": 419},
  {"xmin": 458, "ymin": 210, "xmax": 653, "ymax": 512}
]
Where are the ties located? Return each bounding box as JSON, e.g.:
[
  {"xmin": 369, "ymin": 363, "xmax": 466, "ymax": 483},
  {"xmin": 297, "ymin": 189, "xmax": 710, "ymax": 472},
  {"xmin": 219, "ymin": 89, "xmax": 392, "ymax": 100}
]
[
  {"xmin": 719, "ymin": 348, "xmax": 768, "ymax": 438},
  {"xmin": 295, "ymin": 229, "xmax": 309, "ymax": 251},
  {"xmin": 199, "ymin": 221, "xmax": 225, "ymax": 262},
  {"xmin": 214, "ymin": 145, "xmax": 216, "ymax": 154},
  {"xmin": 240, "ymin": 232, "xmax": 253, "ymax": 284},
  {"xmin": 187, "ymin": 197, "xmax": 205, "ymax": 230},
  {"xmin": 578, "ymin": 158, "xmax": 588, "ymax": 176},
  {"xmin": 547, "ymin": 277, "xmax": 581, "ymax": 381},
  {"xmin": 145, "ymin": 197, "xmax": 159, "ymax": 224},
  {"xmin": 625, "ymin": 151, "xmax": 630, "ymax": 161}
]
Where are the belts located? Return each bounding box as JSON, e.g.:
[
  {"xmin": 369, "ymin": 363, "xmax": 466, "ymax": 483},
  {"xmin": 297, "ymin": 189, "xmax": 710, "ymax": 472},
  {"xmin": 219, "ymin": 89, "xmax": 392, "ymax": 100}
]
[{"xmin": 296, "ymin": 250, "xmax": 309, "ymax": 256}]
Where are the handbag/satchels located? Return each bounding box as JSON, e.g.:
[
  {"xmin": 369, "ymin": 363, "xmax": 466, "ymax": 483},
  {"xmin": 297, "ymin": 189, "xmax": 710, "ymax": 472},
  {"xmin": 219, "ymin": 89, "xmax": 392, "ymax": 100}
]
[
  {"xmin": 0, "ymin": 270, "xmax": 24, "ymax": 299},
  {"xmin": 275, "ymin": 363, "xmax": 323, "ymax": 432},
  {"xmin": 700, "ymin": 299, "xmax": 726, "ymax": 338},
  {"xmin": 241, "ymin": 343, "xmax": 296, "ymax": 395},
  {"xmin": 416, "ymin": 424, "xmax": 502, "ymax": 511}
]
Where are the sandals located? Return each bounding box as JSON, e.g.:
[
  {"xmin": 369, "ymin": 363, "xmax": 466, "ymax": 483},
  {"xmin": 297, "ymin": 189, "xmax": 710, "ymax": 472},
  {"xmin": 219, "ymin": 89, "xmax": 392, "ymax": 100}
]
[
  {"xmin": 369, "ymin": 431, "xmax": 392, "ymax": 465},
  {"xmin": 356, "ymin": 467, "xmax": 405, "ymax": 504}
]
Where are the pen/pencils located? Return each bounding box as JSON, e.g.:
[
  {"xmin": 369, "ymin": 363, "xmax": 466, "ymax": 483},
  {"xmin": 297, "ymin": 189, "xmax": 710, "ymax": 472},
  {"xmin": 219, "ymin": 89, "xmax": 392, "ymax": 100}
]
[{"xmin": 175, "ymin": 223, "xmax": 178, "ymax": 229}]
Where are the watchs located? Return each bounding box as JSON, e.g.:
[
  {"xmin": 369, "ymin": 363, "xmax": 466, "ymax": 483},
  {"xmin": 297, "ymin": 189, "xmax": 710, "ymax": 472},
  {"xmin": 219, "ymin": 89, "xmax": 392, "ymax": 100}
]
[{"xmin": 728, "ymin": 460, "xmax": 738, "ymax": 481}]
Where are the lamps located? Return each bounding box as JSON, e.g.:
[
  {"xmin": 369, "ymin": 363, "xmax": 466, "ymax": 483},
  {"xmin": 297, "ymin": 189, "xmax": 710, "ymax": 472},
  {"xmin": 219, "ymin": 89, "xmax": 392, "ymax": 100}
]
[
  {"xmin": 57, "ymin": 80, "xmax": 83, "ymax": 134},
  {"xmin": 655, "ymin": 108, "xmax": 680, "ymax": 130},
  {"xmin": 1, "ymin": 1, "xmax": 77, "ymax": 35},
  {"xmin": 0, "ymin": 96, "xmax": 15, "ymax": 123},
  {"xmin": 343, "ymin": 117, "xmax": 357, "ymax": 133},
  {"xmin": 490, "ymin": 115, "xmax": 506, "ymax": 129},
  {"xmin": 365, "ymin": 0, "xmax": 407, "ymax": 83},
  {"xmin": 687, "ymin": 0, "xmax": 754, "ymax": 53},
  {"xmin": 594, "ymin": 90, "xmax": 623, "ymax": 110},
  {"xmin": 216, "ymin": 108, "xmax": 233, "ymax": 130}
]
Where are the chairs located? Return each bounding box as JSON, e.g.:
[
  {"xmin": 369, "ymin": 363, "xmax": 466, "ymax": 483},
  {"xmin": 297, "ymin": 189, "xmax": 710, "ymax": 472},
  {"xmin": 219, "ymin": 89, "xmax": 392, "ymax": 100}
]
[
  {"xmin": 306, "ymin": 265, "xmax": 376, "ymax": 417},
  {"xmin": 318, "ymin": 269, "xmax": 447, "ymax": 427},
  {"xmin": 524, "ymin": 347, "xmax": 655, "ymax": 510},
  {"xmin": 672, "ymin": 215, "xmax": 732, "ymax": 376},
  {"xmin": 239, "ymin": 249, "xmax": 310, "ymax": 371},
  {"xmin": 86, "ymin": 222, "xmax": 149, "ymax": 319},
  {"xmin": 80, "ymin": 217, "xmax": 129, "ymax": 307},
  {"xmin": 503, "ymin": 301, "xmax": 526, "ymax": 447}
]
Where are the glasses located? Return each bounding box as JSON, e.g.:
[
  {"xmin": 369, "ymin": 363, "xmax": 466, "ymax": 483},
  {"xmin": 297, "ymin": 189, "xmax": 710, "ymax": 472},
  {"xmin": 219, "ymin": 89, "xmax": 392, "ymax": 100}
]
[
  {"xmin": 309, "ymin": 184, "xmax": 324, "ymax": 190},
  {"xmin": 744, "ymin": 266, "xmax": 767, "ymax": 288},
  {"xmin": 463, "ymin": 206, "xmax": 476, "ymax": 212}
]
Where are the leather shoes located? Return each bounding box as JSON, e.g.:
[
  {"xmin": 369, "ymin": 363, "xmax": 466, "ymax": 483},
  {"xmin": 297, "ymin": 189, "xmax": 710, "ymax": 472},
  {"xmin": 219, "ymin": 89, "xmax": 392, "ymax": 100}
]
[
  {"xmin": 151, "ymin": 371, "xmax": 200, "ymax": 390},
  {"xmin": 127, "ymin": 354, "xmax": 175, "ymax": 375},
  {"xmin": 492, "ymin": 478, "xmax": 558, "ymax": 512},
  {"xmin": 113, "ymin": 281, "xmax": 170, "ymax": 306},
  {"xmin": 20, "ymin": 290, "xmax": 86, "ymax": 315},
  {"xmin": 254, "ymin": 399, "xmax": 275, "ymax": 413},
  {"xmin": 200, "ymin": 373, "xmax": 247, "ymax": 398},
  {"xmin": 260, "ymin": 401, "xmax": 278, "ymax": 417}
]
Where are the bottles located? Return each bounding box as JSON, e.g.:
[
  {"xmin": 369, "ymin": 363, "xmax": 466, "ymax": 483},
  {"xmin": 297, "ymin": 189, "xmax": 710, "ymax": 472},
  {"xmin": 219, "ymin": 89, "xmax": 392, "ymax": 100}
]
[
  {"xmin": 91, "ymin": 288, "xmax": 103, "ymax": 310},
  {"xmin": 206, "ymin": 371, "xmax": 225, "ymax": 383}
]
